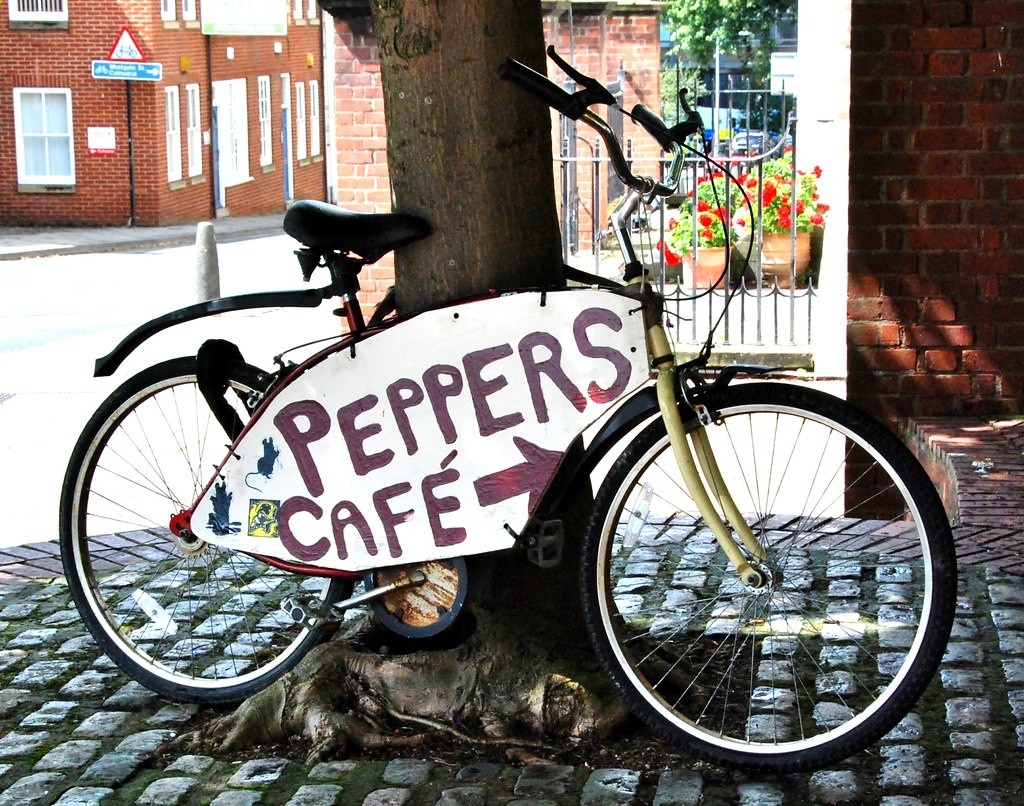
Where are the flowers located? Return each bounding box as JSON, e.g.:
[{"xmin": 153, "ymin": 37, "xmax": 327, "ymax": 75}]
[
  {"xmin": 738, "ymin": 144, "xmax": 831, "ymax": 232},
  {"xmin": 657, "ymin": 171, "xmax": 748, "ymax": 266}
]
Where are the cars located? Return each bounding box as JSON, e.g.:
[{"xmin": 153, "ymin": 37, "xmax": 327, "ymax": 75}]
[{"xmin": 732, "ymin": 131, "xmax": 766, "ymax": 154}]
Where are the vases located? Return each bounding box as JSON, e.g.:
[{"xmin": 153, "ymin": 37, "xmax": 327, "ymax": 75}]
[
  {"xmin": 758, "ymin": 228, "xmax": 809, "ymax": 288},
  {"xmin": 683, "ymin": 246, "xmax": 733, "ymax": 289}
]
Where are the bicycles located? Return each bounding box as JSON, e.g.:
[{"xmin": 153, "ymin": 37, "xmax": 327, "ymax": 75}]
[{"xmin": 56, "ymin": 45, "xmax": 962, "ymax": 781}]
